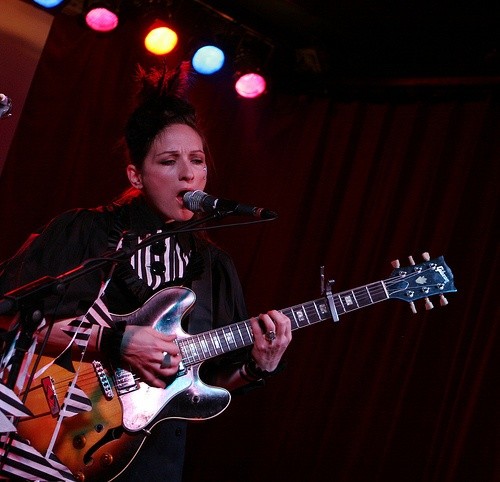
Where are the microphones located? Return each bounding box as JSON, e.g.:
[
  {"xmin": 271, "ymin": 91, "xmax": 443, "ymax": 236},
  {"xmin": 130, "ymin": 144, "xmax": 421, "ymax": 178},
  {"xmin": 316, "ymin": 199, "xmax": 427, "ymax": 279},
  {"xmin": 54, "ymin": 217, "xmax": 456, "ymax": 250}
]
[{"xmin": 184, "ymin": 189, "xmax": 278, "ymax": 220}]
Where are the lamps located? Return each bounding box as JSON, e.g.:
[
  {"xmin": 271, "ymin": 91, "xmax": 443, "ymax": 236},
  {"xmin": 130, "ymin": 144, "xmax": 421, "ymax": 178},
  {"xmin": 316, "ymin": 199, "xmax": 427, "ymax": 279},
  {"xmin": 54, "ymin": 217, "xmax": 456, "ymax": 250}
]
[
  {"xmin": 235, "ymin": 36, "xmax": 267, "ymax": 100},
  {"xmin": 83, "ymin": 1, "xmax": 124, "ymax": 34},
  {"xmin": 143, "ymin": 0, "xmax": 181, "ymax": 57},
  {"xmin": 185, "ymin": 28, "xmax": 226, "ymax": 75}
]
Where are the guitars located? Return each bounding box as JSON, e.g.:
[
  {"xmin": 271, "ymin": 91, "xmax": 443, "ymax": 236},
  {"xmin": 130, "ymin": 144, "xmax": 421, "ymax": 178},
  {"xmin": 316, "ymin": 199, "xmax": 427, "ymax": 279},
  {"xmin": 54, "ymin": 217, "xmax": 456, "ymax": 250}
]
[{"xmin": 13, "ymin": 251, "xmax": 458, "ymax": 482}]
